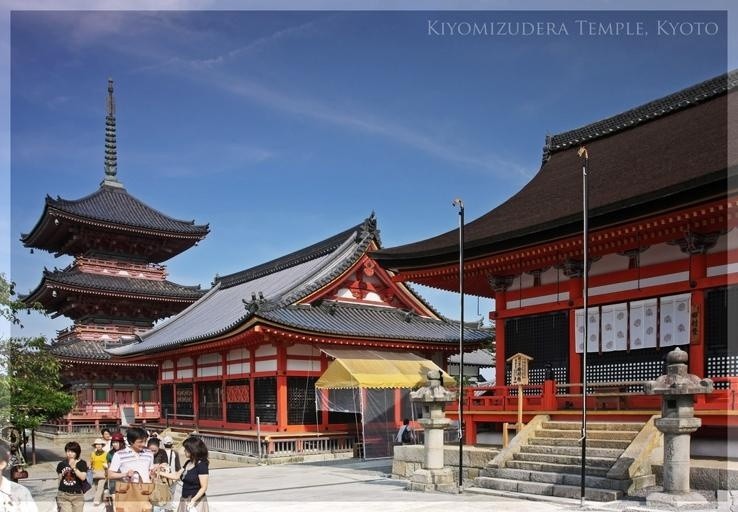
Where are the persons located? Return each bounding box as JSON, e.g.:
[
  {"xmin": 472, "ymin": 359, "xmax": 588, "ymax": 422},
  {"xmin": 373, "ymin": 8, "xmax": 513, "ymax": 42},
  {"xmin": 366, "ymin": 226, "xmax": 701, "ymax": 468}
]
[
  {"xmin": 0, "ymin": 439, "xmax": 40, "ymax": 512},
  {"xmin": 153, "ymin": 436, "xmax": 209, "ymax": 512},
  {"xmin": 89, "ymin": 425, "xmax": 180, "ymax": 512},
  {"xmin": 393, "ymin": 418, "xmax": 416, "ymax": 444},
  {"xmin": 55, "ymin": 442, "xmax": 93, "ymax": 512}
]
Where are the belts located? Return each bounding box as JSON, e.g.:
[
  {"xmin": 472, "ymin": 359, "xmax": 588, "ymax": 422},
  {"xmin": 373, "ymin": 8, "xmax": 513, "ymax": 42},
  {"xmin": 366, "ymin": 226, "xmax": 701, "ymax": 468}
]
[{"xmin": 58, "ymin": 487, "xmax": 82, "ymax": 494}]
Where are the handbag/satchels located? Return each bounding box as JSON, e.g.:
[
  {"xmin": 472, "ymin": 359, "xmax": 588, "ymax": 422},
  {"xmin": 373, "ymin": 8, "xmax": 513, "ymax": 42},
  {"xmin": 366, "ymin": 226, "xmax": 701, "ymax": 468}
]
[
  {"xmin": 113, "ymin": 470, "xmax": 155, "ymax": 512},
  {"xmin": 150, "ymin": 466, "xmax": 172, "ymax": 506},
  {"xmin": 92, "ymin": 470, "xmax": 106, "ymax": 479}
]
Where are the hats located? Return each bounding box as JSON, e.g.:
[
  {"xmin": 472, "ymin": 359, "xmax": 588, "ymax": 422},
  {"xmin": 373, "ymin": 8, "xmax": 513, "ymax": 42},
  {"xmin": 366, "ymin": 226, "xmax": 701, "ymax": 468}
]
[
  {"xmin": 91, "ymin": 439, "xmax": 106, "ymax": 447},
  {"xmin": 112, "ymin": 433, "xmax": 123, "ymax": 441},
  {"xmin": 164, "ymin": 436, "xmax": 173, "ymax": 446}
]
